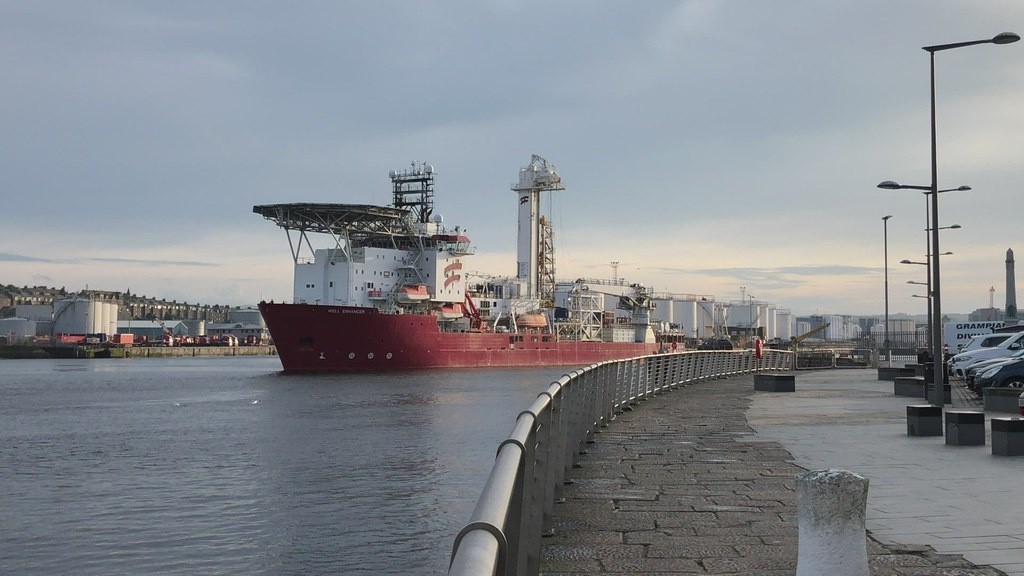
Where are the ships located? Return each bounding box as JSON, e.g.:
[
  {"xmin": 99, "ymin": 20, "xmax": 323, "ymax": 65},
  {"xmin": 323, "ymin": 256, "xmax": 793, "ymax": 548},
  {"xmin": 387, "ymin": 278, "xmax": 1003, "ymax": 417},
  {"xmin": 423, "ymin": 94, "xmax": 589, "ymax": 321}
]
[{"xmin": 252, "ymin": 153, "xmax": 686, "ymax": 378}]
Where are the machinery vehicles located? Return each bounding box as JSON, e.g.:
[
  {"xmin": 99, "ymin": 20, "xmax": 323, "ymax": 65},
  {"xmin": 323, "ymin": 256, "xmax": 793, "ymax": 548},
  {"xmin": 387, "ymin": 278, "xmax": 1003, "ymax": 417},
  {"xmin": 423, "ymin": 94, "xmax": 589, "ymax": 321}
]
[{"xmin": 766, "ymin": 321, "xmax": 831, "ymax": 352}]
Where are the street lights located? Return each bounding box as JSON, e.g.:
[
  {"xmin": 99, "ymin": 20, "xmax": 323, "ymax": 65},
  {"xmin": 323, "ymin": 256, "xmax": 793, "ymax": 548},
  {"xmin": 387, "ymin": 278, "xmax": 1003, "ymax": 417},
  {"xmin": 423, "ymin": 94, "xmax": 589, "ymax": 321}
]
[
  {"xmin": 877, "ymin": 182, "xmax": 972, "ymax": 361},
  {"xmin": 882, "ymin": 215, "xmax": 893, "ymax": 361},
  {"xmin": 747, "ymin": 293, "xmax": 755, "ymax": 348},
  {"xmin": 921, "ymin": 31, "xmax": 1022, "ymax": 407}
]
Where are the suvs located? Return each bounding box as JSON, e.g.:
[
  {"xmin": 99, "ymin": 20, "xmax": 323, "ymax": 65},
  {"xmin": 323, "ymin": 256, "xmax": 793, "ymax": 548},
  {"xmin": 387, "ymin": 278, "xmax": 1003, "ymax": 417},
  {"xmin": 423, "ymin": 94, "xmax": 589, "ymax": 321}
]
[{"xmin": 697, "ymin": 338, "xmax": 733, "ymax": 355}]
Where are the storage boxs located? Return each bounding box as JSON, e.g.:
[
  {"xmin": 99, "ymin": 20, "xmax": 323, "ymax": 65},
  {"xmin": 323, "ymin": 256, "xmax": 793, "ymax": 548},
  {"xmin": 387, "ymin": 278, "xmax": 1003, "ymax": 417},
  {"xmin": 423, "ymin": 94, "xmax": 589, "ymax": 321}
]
[{"xmin": 113, "ymin": 333, "xmax": 133, "ymax": 343}]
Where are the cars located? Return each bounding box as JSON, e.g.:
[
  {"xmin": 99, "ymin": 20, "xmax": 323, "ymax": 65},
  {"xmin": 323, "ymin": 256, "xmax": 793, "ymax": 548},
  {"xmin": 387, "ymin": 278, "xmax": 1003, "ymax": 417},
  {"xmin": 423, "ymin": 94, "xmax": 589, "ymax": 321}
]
[{"xmin": 946, "ymin": 324, "xmax": 1024, "ymax": 412}]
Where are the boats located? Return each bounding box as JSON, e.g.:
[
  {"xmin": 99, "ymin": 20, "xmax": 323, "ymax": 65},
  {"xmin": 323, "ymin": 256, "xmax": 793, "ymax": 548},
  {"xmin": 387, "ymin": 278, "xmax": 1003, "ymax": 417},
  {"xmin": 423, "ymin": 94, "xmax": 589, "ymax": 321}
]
[{"xmin": 517, "ymin": 313, "xmax": 549, "ymax": 330}]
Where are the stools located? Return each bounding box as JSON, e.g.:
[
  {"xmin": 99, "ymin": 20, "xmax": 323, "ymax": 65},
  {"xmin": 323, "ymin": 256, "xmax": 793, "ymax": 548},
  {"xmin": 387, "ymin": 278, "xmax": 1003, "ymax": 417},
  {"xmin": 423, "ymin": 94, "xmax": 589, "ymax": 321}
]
[
  {"xmin": 945, "ymin": 411, "xmax": 985, "ymax": 446},
  {"xmin": 991, "ymin": 416, "xmax": 1024, "ymax": 457},
  {"xmin": 906, "ymin": 404, "xmax": 943, "ymax": 437}
]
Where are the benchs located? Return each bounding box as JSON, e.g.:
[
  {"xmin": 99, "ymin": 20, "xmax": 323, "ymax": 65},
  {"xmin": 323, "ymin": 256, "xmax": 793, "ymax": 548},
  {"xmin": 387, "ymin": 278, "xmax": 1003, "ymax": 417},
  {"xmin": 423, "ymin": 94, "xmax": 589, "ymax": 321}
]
[
  {"xmin": 905, "ymin": 363, "xmax": 923, "ymax": 376},
  {"xmin": 894, "ymin": 377, "xmax": 924, "ymax": 397},
  {"xmin": 878, "ymin": 367, "xmax": 915, "ymax": 381},
  {"xmin": 754, "ymin": 374, "xmax": 795, "ymax": 392}
]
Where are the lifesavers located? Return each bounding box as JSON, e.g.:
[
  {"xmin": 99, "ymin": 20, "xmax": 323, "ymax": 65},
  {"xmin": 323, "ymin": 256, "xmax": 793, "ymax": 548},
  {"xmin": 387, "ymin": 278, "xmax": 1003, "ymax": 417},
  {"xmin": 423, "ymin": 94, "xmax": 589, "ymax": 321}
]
[
  {"xmin": 756, "ymin": 340, "xmax": 763, "ymax": 358},
  {"xmin": 672, "ymin": 343, "xmax": 677, "ymax": 349}
]
